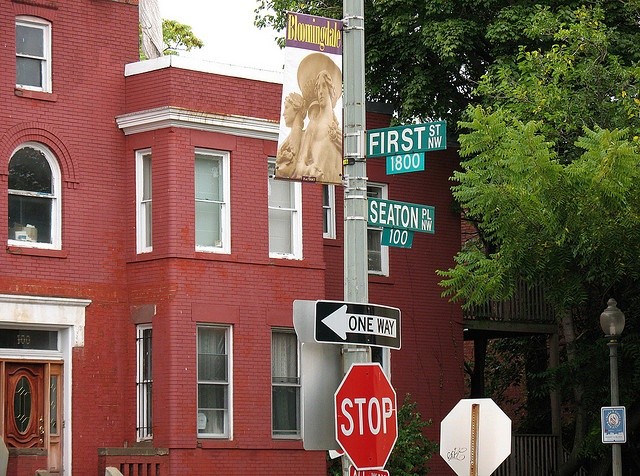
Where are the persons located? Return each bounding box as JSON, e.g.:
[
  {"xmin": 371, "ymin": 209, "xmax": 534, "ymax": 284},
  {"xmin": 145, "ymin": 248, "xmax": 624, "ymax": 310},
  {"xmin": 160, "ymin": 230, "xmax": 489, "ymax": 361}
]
[
  {"xmin": 275, "ymin": 92, "xmax": 308, "ymax": 179},
  {"xmin": 296, "ymin": 69, "xmax": 342, "ymax": 182}
]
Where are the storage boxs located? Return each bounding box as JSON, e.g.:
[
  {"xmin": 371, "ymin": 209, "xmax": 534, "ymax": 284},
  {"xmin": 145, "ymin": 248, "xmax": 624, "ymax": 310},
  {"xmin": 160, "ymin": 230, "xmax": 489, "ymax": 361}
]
[{"xmin": 8, "ymin": 226, "xmax": 38, "ymax": 241}]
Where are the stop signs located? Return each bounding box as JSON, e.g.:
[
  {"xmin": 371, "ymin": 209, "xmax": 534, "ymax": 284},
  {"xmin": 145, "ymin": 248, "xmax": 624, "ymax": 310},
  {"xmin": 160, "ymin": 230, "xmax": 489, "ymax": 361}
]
[{"xmin": 334, "ymin": 362, "xmax": 399, "ymax": 472}]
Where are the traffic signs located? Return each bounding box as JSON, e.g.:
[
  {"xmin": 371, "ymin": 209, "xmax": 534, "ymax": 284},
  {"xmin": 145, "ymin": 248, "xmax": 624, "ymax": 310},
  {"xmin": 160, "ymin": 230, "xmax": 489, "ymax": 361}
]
[
  {"xmin": 367, "ymin": 197, "xmax": 435, "ymax": 249},
  {"xmin": 366, "ymin": 121, "xmax": 447, "ymax": 175},
  {"xmin": 599, "ymin": 297, "xmax": 626, "ymax": 476},
  {"xmin": 314, "ymin": 299, "xmax": 402, "ymax": 350}
]
